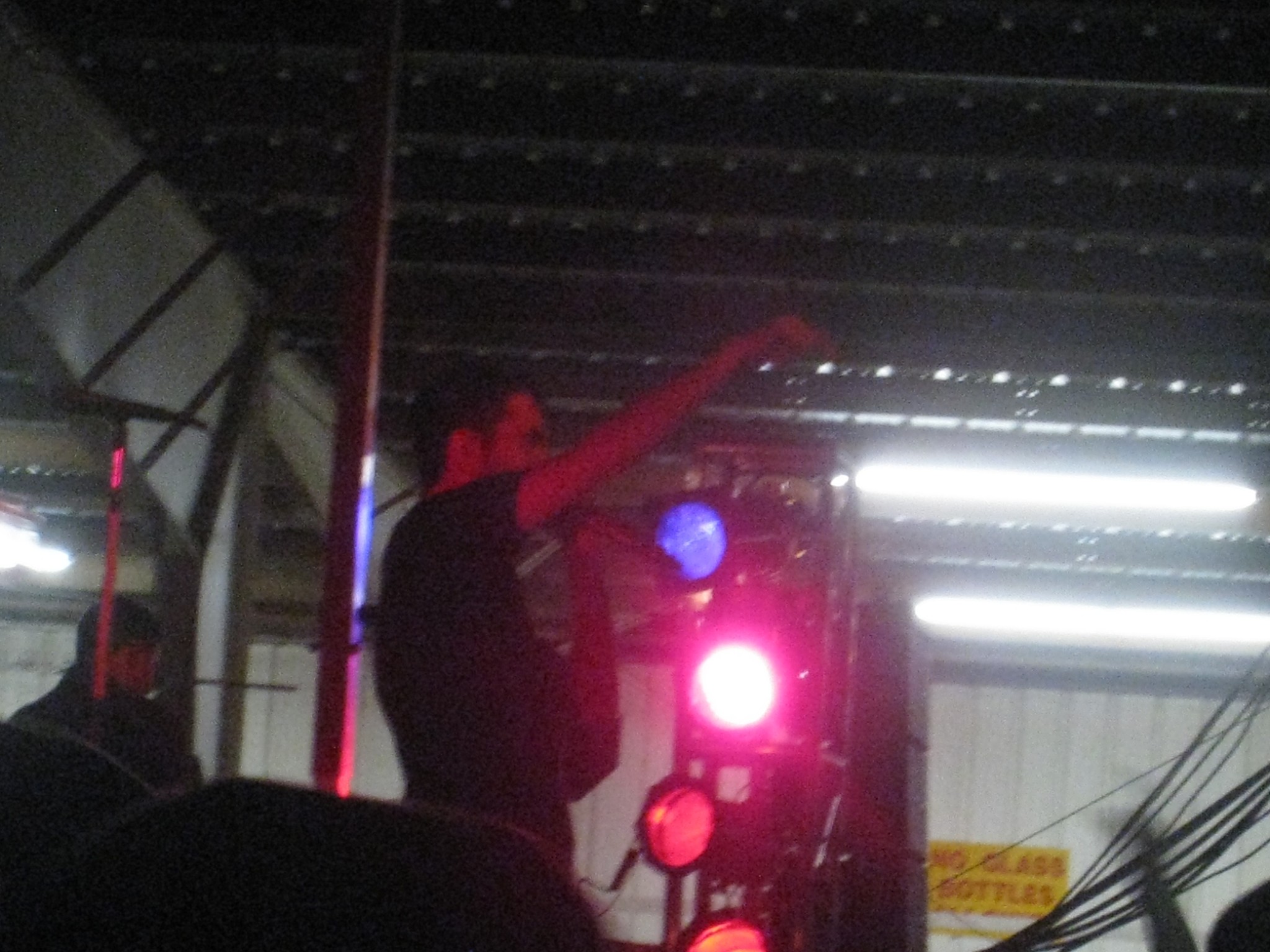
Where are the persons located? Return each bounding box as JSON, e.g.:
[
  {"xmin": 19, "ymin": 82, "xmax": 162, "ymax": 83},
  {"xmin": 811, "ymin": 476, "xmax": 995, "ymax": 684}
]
[
  {"xmin": 373, "ymin": 312, "xmax": 838, "ymax": 879},
  {"xmin": 5, "ymin": 593, "xmax": 201, "ymax": 788}
]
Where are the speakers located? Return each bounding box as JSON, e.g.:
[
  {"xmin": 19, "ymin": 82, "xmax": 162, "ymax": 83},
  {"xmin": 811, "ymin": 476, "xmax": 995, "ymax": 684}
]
[{"xmin": 721, "ymin": 599, "xmax": 929, "ymax": 952}]
